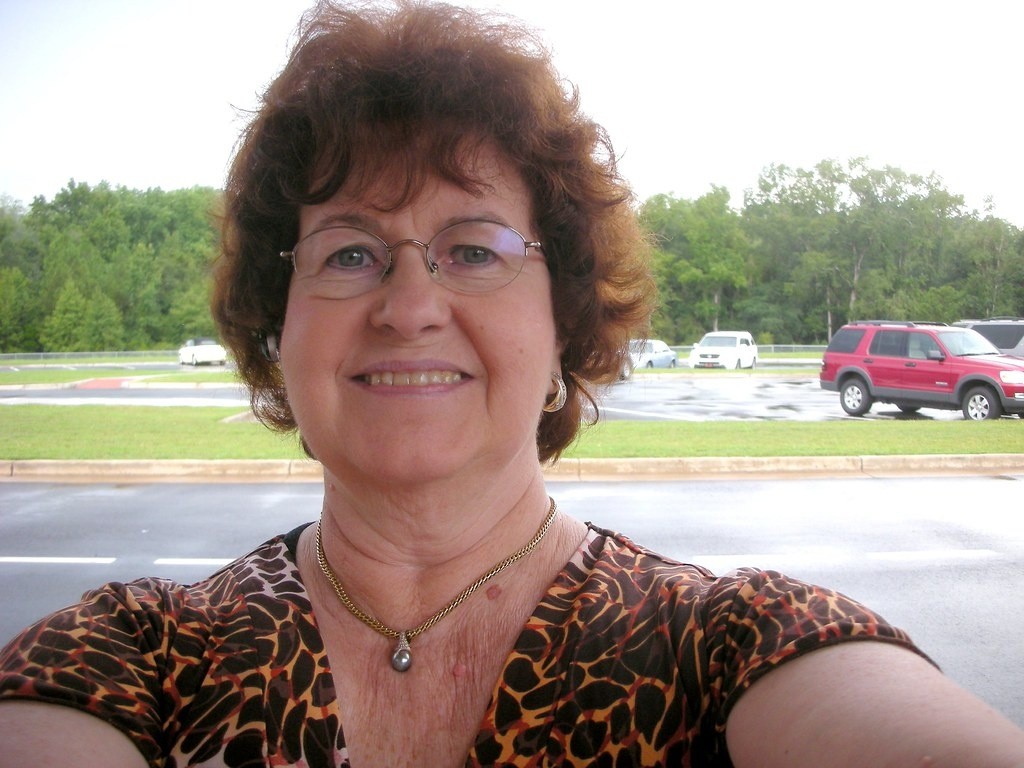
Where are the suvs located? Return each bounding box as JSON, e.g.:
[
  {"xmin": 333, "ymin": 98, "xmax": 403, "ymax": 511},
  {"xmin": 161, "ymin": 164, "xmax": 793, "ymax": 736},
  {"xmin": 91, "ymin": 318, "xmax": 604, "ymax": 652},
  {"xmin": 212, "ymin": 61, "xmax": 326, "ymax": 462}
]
[
  {"xmin": 820, "ymin": 320, "xmax": 1024, "ymax": 421},
  {"xmin": 952, "ymin": 316, "xmax": 1024, "ymax": 357}
]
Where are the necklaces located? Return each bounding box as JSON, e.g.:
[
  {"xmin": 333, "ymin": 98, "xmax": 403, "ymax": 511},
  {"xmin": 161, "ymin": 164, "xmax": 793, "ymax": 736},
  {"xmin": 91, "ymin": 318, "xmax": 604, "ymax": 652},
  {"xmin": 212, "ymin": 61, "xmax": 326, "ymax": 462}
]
[{"xmin": 312, "ymin": 494, "xmax": 553, "ymax": 670}]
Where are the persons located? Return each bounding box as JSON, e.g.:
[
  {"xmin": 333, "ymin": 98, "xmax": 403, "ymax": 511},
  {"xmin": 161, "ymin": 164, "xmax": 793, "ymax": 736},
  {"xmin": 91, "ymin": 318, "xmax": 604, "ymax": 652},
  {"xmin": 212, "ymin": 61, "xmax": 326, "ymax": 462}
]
[{"xmin": 1, "ymin": 0, "xmax": 1023, "ymax": 767}]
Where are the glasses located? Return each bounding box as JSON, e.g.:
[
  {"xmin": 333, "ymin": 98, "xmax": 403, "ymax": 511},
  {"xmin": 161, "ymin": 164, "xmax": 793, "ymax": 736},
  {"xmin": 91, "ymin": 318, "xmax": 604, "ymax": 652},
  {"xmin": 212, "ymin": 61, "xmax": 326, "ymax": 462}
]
[{"xmin": 278, "ymin": 217, "xmax": 543, "ymax": 295}]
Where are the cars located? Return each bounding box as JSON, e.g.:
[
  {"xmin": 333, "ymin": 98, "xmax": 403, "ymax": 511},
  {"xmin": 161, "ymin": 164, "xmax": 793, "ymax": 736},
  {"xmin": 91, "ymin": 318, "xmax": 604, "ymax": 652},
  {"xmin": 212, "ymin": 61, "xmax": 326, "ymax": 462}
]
[
  {"xmin": 689, "ymin": 329, "xmax": 758, "ymax": 370},
  {"xmin": 178, "ymin": 338, "xmax": 227, "ymax": 366},
  {"xmin": 588, "ymin": 340, "xmax": 677, "ymax": 380}
]
